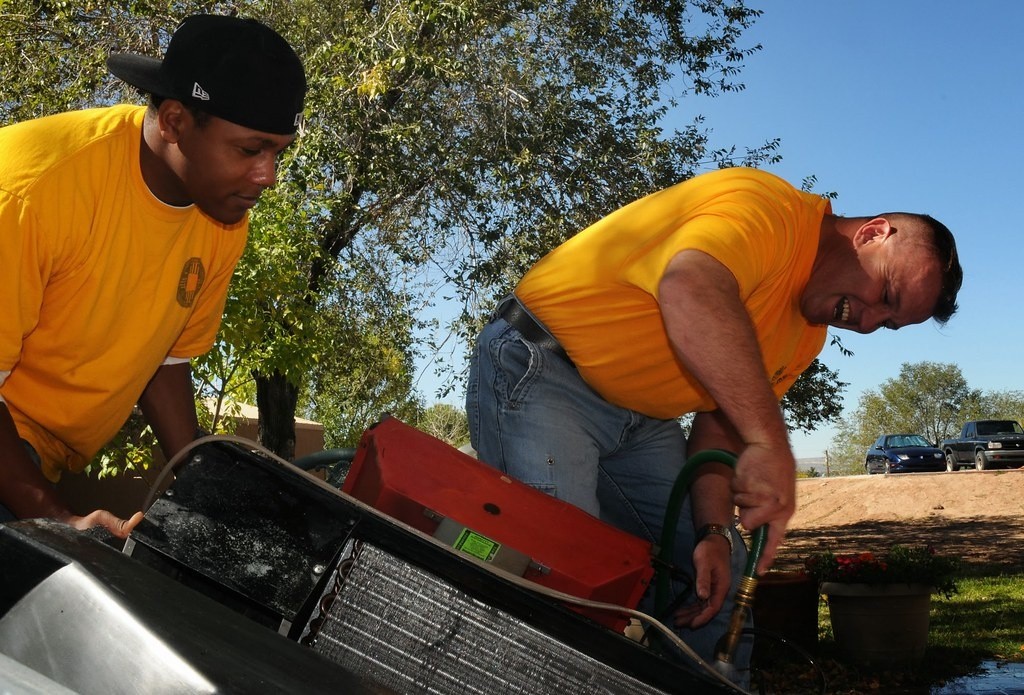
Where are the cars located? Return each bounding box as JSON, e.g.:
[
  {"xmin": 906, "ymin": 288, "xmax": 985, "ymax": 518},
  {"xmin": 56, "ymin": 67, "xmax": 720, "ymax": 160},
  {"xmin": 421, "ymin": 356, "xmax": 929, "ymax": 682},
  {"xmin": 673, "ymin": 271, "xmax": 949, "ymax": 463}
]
[{"xmin": 864, "ymin": 434, "xmax": 948, "ymax": 474}]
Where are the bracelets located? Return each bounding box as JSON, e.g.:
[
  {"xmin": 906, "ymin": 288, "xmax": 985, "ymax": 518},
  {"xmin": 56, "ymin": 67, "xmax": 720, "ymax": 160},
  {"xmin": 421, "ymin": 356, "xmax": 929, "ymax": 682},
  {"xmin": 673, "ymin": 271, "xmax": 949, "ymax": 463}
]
[{"xmin": 695, "ymin": 523, "xmax": 734, "ymax": 559}]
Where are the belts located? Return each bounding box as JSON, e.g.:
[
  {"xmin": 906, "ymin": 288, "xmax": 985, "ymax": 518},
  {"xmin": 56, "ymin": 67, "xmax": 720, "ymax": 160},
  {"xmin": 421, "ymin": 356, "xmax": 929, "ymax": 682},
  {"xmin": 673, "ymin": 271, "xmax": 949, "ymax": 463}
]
[{"xmin": 500, "ymin": 295, "xmax": 576, "ymax": 368}]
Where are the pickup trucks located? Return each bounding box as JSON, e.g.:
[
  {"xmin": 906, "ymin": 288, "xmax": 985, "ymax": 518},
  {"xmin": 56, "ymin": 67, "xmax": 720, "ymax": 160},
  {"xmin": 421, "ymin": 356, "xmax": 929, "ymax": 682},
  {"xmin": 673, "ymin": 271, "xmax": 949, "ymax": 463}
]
[{"xmin": 939, "ymin": 420, "xmax": 1024, "ymax": 471}]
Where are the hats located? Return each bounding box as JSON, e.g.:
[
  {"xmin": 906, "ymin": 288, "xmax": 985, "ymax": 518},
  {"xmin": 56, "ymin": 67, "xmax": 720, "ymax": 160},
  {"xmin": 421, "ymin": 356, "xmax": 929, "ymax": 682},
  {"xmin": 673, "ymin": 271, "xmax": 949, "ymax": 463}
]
[{"xmin": 107, "ymin": 14, "xmax": 307, "ymax": 134}]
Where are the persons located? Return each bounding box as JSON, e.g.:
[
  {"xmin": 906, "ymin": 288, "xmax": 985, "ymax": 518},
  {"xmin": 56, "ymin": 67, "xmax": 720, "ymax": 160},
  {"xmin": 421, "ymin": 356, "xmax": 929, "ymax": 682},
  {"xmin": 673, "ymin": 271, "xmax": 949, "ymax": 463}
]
[
  {"xmin": 464, "ymin": 167, "xmax": 964, "ymax": 695},
  {"xmin": 1, "ymin": 14, "xmax": 307, "ymax": 540}
]
